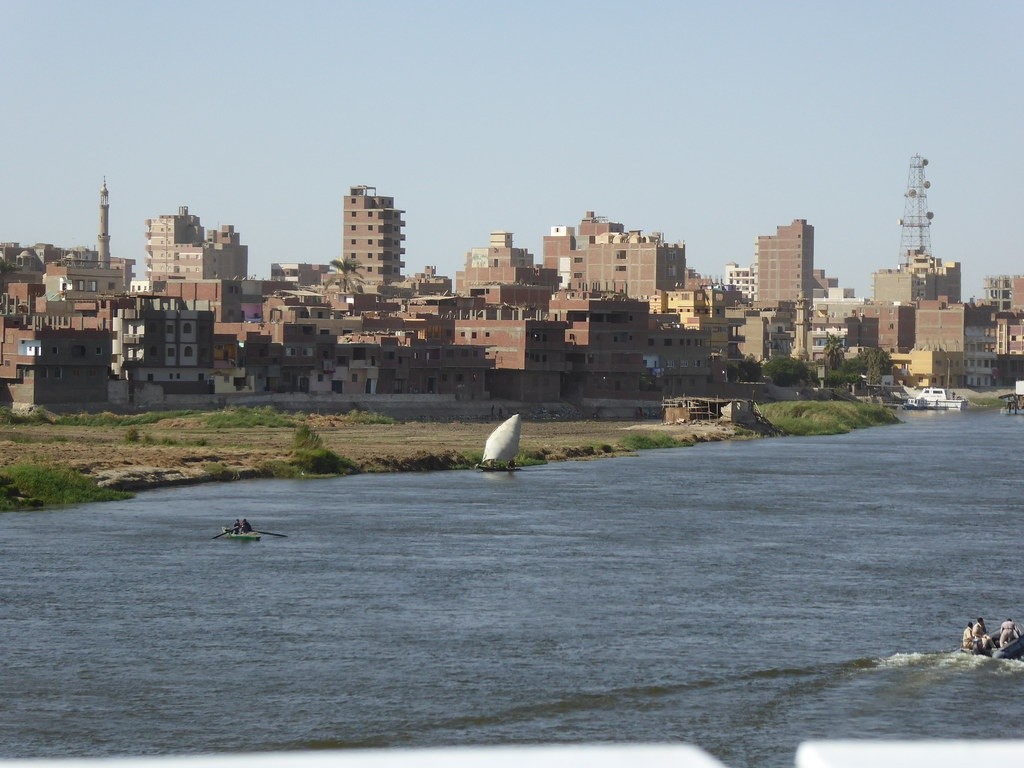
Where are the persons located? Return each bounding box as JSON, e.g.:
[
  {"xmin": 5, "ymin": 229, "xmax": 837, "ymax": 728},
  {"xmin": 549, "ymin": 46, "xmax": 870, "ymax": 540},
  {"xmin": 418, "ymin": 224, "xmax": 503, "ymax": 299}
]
[
  {"xmin": 999, "ymin": 617, "xmax": 1020, "ymax": 648},
  {"xmin": 972, "ymin": 617, "xmax": 996, "ymax": 649},
  {"xmin": 962, "ymin": 621, "xmax": 981, "ymax": 653},
  {"xmin": 233, "ymin": 518, "xmax": 252, "ymax": 534}
]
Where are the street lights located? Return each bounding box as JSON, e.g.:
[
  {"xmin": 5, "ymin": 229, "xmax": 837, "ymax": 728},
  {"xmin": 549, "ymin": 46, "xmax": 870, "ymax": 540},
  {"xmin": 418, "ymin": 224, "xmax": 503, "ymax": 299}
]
[{"xmin": 947, "ymin": 358, "xmax": 950, "ymax": 389}]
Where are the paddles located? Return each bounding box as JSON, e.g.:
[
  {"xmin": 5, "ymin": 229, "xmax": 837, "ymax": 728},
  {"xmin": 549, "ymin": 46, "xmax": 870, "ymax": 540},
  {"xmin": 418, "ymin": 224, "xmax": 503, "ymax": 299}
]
[
  {"xmin": 211, "ymin": 526, "xmax": 239, "ymax": 539},
  {"xmin": 250, "ymin": 529, "xmax": 289, "ymax": 537}
]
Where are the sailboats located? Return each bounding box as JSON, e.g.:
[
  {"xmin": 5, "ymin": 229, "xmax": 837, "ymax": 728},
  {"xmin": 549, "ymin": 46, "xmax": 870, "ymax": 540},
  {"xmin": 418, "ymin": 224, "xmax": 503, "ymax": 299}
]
[{"xmin": 478, "ymin": 414, "xmax": 521, "ymax": 471}]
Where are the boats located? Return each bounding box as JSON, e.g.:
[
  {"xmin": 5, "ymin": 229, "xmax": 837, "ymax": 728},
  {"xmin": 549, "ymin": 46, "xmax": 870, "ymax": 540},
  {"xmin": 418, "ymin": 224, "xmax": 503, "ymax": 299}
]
[
  {"xmin": 222, "ymin": 526, "xmax": 261, "ymax": 541},
  {"xmin": 915, "ymin": 386, "xmax": 969, "ymax": 410},
  {"xmin": 956, "ymin": 628, "xmax": 1024, "ymax": 659},
  {"xmin": 903, "ymin": 397, "xmax": 930, "ymax": 410}
]
